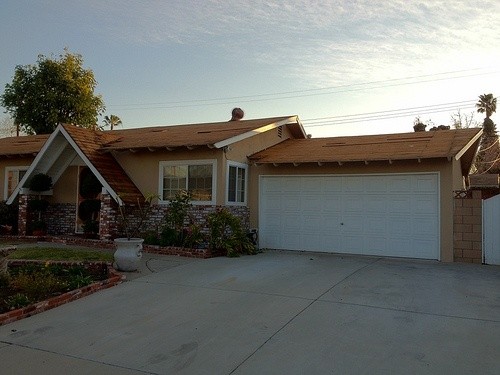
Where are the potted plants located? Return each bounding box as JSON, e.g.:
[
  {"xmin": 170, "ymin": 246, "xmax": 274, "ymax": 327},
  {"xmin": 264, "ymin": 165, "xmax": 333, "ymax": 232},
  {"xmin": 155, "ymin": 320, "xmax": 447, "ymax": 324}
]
[
  {"xmin": 113, "ymin": 193, "xmax": 160, "ymax": 271},
  {"xmin": 78, "ymin": 168, "xmax": 100, "ymax": 239},
  {"xmin": 28, "ymin": 173, "xmax": 53, "ymax": 236}
]
